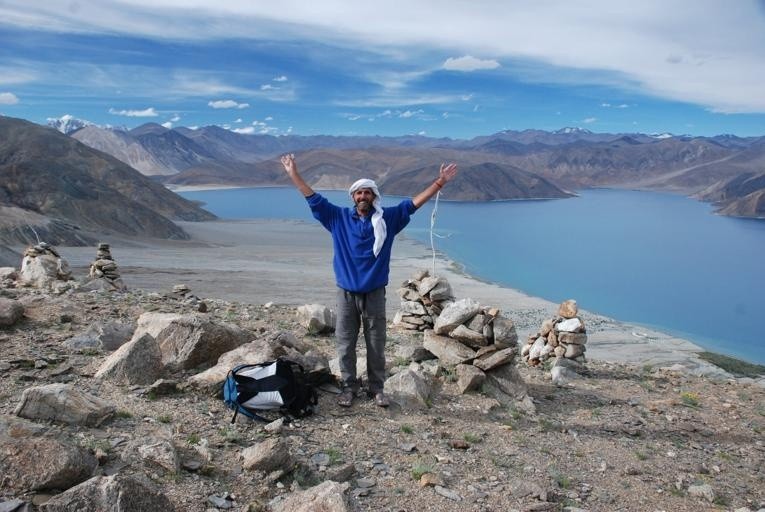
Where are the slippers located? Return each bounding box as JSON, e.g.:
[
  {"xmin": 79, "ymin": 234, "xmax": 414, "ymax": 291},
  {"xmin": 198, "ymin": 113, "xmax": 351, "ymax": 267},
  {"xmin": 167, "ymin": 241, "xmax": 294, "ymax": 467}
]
[
  {"xmin": 337, "ymin": 390, "xmax": 356, "ymax": 408},
  {"xmin": 373, "ymin": 391, "xmax": 390, "ymax": 407}
]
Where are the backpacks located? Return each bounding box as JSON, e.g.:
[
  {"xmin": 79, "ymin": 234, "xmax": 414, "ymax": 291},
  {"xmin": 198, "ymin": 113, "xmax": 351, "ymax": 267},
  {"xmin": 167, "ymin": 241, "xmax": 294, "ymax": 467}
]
[{"xmin": 221, "ymin": 358, "xmax": 318, "ymax": 422}]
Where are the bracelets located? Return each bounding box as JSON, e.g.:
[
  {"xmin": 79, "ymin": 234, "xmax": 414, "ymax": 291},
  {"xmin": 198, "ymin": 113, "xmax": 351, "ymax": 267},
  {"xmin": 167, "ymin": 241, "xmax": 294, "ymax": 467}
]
[{"xmin": 432, "ymin": 180, "xmax": 445, "ymax": 190}]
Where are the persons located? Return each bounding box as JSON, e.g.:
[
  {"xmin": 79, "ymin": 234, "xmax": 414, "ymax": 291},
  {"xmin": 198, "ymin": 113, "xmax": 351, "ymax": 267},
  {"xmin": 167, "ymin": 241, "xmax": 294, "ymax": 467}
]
[{"xmin": 279, "ymin": 151, "xmax": 459, "ymax": 408}]
